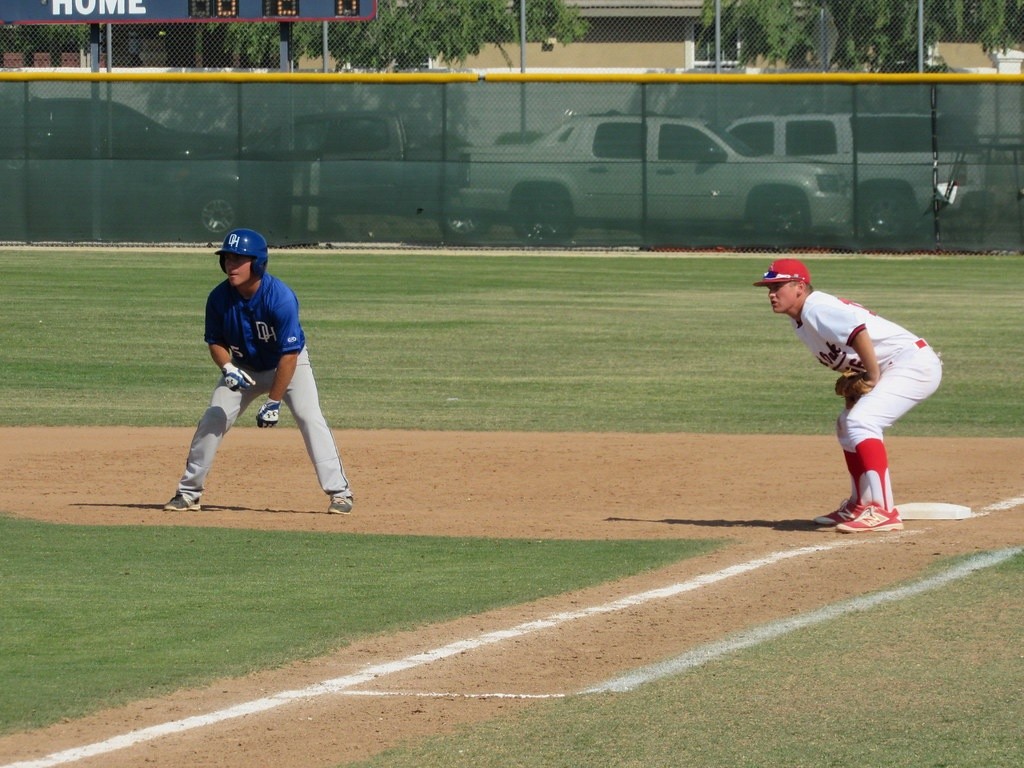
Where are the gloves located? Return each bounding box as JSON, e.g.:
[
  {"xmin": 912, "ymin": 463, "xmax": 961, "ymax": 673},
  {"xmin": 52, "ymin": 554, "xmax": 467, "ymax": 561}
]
[
  {"xmin": 256, "ymin": 398, "xmax": 283, "ymax": 427},
  {"xmin": 222, "ymin": 362, "xmax": 257, "ymax": 393}
]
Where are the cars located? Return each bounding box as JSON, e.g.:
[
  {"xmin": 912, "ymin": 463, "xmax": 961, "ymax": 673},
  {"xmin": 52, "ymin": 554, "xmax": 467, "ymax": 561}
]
[
  {"xmin": 451, "ymin": 111, "xmax": 852, "ymax": 246},
  {"xmin": 1, "ymin": 99, "xmax": 210, "ymax": 242},
  {"xmin": 169, "ymin": 111, "xmax": 498, "ymax": 242}
]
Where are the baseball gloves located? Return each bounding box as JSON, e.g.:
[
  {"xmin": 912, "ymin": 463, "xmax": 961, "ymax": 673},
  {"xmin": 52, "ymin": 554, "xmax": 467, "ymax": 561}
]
[{"xmin": 834, "ymin": 368, "xmax": 875, "ymax": 399}]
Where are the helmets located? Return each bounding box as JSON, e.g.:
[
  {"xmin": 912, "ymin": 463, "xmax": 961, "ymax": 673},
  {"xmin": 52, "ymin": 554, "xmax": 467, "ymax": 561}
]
[{"xmin": 215, "ymin": 228, "xmax": 269, "ymax": 279}]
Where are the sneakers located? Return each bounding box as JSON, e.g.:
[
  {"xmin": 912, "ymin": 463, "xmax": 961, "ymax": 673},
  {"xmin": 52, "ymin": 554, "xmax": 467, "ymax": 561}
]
[
  {"xmin": 812, "ymin": 497, "xmax": 869, "ymax": 526},
  {"xmin": 163, "ymin": 497, "xmax": 202, "ymax": 513},
  {"xmin": 327, "ymin": 495, "xmax": 356, "ymax": 516},
  {"xmin": 836, "ymin": 504, "xmax": 905, "ymax": 533}
]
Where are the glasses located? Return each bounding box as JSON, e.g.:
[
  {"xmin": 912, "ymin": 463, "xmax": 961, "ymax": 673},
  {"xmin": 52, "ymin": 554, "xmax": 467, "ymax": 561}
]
[{"xmin": 762, "ymin": 270, "xmax": 806, "ymax": 281}]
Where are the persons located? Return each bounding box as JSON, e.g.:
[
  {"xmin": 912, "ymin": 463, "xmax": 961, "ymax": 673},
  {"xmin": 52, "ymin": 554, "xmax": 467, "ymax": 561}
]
[
  {"xmin": 163, "ymin": 228, "xmax": 354, "ymax": 514},
  {"xmin": 751, "ymin": 258, "xmax": 942, "ymax": 534}
]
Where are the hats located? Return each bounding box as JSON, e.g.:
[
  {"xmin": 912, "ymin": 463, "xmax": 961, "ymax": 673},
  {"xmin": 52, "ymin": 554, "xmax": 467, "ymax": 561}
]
[{"xmin": 752, "ymin": 259, "xmax": 812, "ymax": 287}]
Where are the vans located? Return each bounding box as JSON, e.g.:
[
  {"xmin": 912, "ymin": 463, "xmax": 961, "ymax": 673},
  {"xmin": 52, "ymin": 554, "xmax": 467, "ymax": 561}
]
[{"xmin": 726, "ymin": 108, "xmax": 991, "ymax": 245}]
